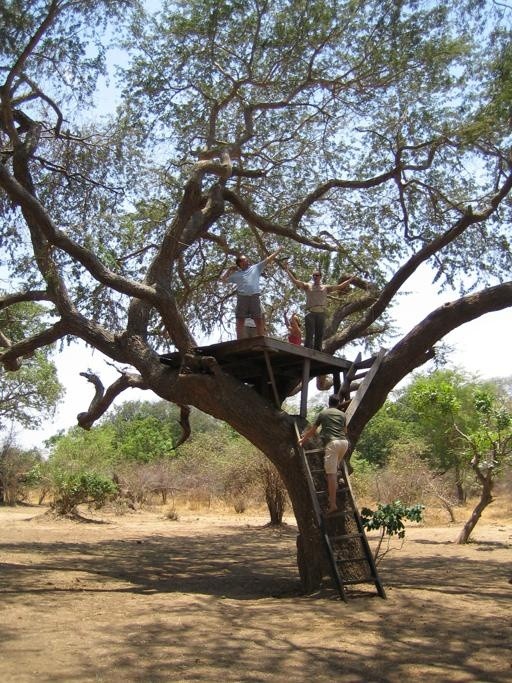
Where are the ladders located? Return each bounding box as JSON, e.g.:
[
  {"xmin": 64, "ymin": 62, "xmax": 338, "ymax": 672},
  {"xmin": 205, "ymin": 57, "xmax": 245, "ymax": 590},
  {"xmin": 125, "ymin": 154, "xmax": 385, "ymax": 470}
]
[
  {"xmin": 293, "ymin": 421, "xmax": 387, "ymax": 601},
  {"xmin": 332, "ymin": 348, "xmax": 386, "ymax": 426}
]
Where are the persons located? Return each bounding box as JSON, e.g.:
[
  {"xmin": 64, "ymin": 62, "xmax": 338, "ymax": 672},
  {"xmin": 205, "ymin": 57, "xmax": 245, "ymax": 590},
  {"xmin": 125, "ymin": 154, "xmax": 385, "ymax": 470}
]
[
  {"xmin": 285, "ymin": 260, "xmax": 356, "ymax": 351},
  {"xmin": 242, "ymin": 294, "xmax": 267, "ymax": 338},
  {"xmin": 296, "ymin": 393, "xmax": 348, "ymax": 514},
  {"xmin": 284, "ymin": 310, "xmax": 302, "ymax": 345},
  {"xmin": 224, "ymin": 243, "xmax": 284, "ymax": 340}
]
